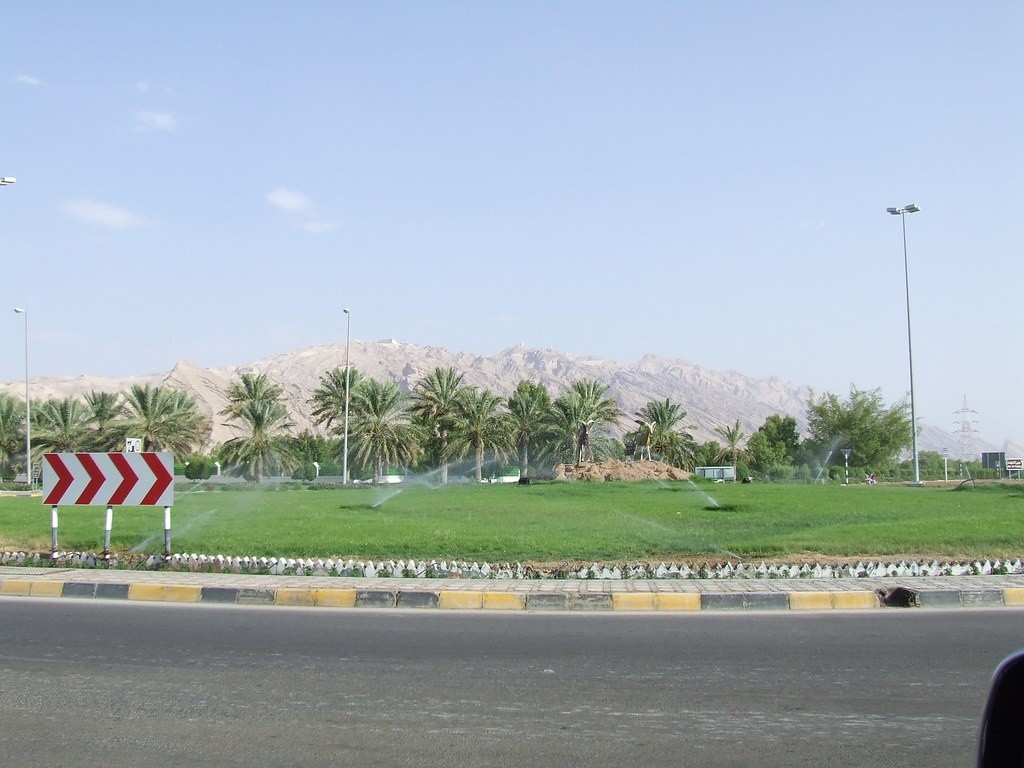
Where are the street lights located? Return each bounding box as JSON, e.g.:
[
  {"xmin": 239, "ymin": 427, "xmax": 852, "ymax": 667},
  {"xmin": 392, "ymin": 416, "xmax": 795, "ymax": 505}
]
[
  {"xmin": 999, "ymin": 449, "xmax": 1001, "ymax": 465},
  {"xmin": 343, "ymin": 307, "xmax": 351, "ymax": 483},
  {"xmin": 886, "ymin": 204, "xmax": 921, "ymax": 481},
  {"xmin": 15, "ymin": 307, "xmax": 31, "ymax": 487},
  {"xmin": 942, "ymin": 457, "xmax": 947, "ymax": 483}
]
